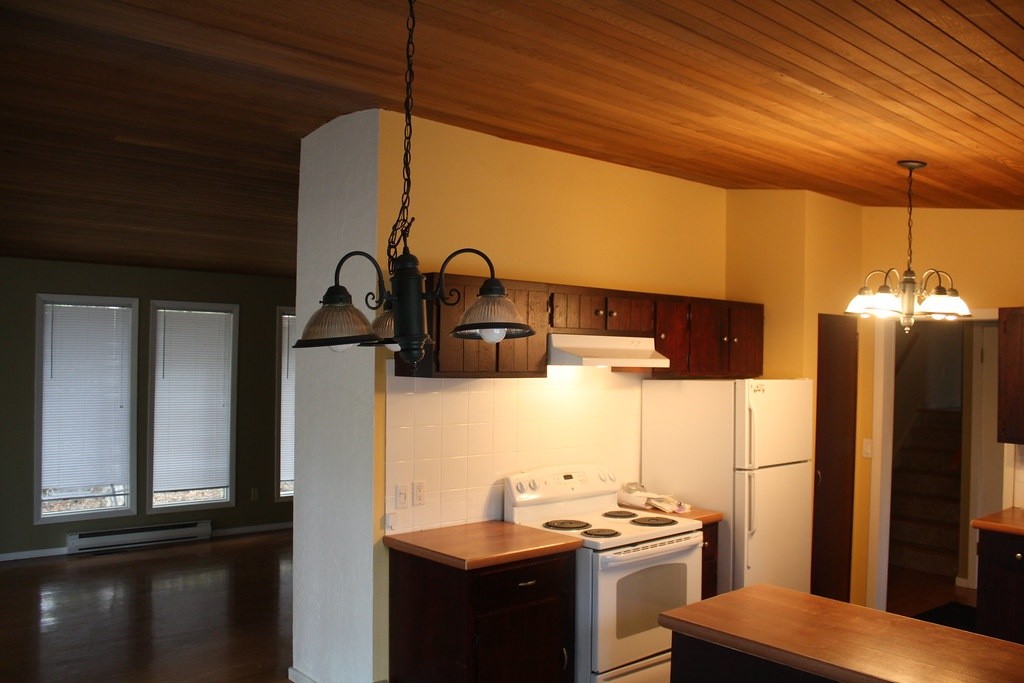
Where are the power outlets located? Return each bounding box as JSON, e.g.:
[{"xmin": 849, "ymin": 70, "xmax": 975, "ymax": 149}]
[{"xmin": 412, "ymin": 480, "xmax": 426, "ymax": 506}]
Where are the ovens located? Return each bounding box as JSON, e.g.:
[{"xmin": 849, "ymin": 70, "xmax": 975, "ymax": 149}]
[{"xmin": 575, "ymin": 530, "xmax": 703, "ymax": 683}]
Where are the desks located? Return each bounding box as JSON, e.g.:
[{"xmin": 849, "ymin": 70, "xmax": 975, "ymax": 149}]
[{"xmin": 657, "ymin": 581, "xmax": 1024, "ymax": 683}]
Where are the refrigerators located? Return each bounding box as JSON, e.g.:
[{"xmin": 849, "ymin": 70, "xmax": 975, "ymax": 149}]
[{"xmin": 641, "ymin": 377, "xmax": 815, "ymax": 603}]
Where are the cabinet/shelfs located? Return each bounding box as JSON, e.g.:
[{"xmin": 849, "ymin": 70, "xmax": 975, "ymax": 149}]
[
  {"xmin": 997, "ymin": 305, "xmax": 1023, "ymax": 447},
  {"xmin": 381, "ymin": 552, "xmax": 582, "ymax": 683},
  {"xmin": 395, "ymin": 271, "xmax": 764, "ymax": 379},
  {"xmin": 971, "ymin": 530, "xmax": 1024, "ymax": 643},
  {"xmin": 702, "ymin": 523, "xmax": 719, "ymax": 601}
]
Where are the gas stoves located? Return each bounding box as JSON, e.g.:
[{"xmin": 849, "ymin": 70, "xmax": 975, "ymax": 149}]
[{"xmin": 503, "ymin": 465, "xmax": 703, "ymax": 550}]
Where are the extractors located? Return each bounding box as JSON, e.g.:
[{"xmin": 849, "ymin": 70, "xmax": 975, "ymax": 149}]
[{"xmin": 547, "ymin": 333, "xmax": 670, "ymax": 369}]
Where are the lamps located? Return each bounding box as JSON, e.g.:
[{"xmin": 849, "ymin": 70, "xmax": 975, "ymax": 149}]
[
  {"xmin": 844, "ymin": 160, "xmax": 974, "ymax": 335},
  {"xmin": 291, "ymin": 1, "xmax": 535, "ymax": 353}
]
